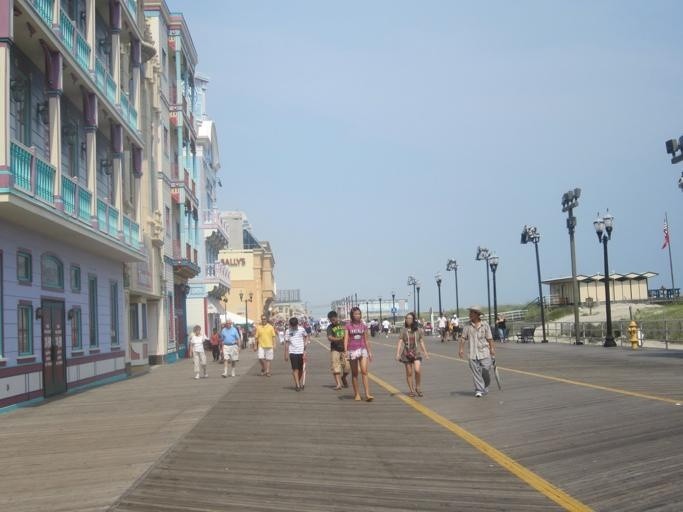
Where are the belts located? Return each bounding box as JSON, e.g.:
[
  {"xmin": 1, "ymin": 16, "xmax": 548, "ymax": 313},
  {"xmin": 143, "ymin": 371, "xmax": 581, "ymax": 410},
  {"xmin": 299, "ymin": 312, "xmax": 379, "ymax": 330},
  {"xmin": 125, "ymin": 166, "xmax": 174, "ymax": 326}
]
[{"xmin": 225, "ymin": 343, "xmax": 237, "ymax": 346}]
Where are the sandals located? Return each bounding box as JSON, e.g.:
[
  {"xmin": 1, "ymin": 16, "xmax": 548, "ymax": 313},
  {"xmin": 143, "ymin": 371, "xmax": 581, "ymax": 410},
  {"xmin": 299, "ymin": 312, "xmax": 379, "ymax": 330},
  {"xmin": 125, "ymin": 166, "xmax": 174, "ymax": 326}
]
[{"xmin": 409, "ymin": 388, "xmax": 424, "ymax": 397}]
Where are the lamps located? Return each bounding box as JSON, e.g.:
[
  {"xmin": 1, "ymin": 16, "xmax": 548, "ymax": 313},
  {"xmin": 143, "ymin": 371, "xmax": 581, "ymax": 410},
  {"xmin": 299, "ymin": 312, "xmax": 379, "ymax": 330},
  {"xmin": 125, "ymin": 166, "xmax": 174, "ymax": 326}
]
[
  {"xmin": 33, "ymin": 305, "xmax": 48, "ymax": 320},
  {"xmin": 36, "ymin": 100, "xmax": 49, "ymax": 125},
  {"xmin": 65, "ymin": 307, "xmax": 78, "ymax": 320},
  {"xmin": 99, "ymin": 157, "xmax": 112, "ymax": 175},
  {"xmin": 99, "ymin": 36, "xmax": 111, "ymax": 55}
]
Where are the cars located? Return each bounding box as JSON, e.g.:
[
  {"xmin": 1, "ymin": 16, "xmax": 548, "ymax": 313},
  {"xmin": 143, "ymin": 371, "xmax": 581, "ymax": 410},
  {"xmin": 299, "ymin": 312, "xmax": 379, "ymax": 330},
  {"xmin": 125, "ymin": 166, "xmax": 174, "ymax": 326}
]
[{"xmin": 309, "ymin": 320, "xmax": 318, "ymax": 325}]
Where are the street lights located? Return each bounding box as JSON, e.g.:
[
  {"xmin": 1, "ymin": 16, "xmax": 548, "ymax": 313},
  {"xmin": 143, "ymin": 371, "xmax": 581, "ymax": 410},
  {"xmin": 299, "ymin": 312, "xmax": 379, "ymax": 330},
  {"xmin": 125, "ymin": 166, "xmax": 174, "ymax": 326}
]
[
  {"xmin": 487, "ymin": 251, "xmax": 500, "ymax": 341},
  {"xmin": 329, "ymin": 292, "xmax": 361, "ymax": 321},
  {"xmin": 377, "ymin": 297, "xmax": 383, "ymax": 326},
  {"xmin": 519, "ymin": 224, "xmax": 549, "ymax": 345},
  {"xmin": 237, "ymin": 289, "xmax": 254, "ymax": 347},
  {"xmin": 406, "ymin": 275, "xmax": 416, "ymax": 316},
  {"xmin": 664, "ymin": 136, "xmax": 682, "ymax": 192},
  {"xmin": 593, "ymin": 208, "xmax": 618, "ymax": 348},
  {"xmin": 432, "ymin": 270, "xmax": 443, "ymax": 318},
  {"xmin": 559, "ymin": 187, "xmax": 585, "ymax": 345},
  {"xmin": 390, "ymin": 292, "xmax": 395, "ymax": 325},
  {"xmin": 366, "ymin": 301, "xmax": 370, "ymax": 323},
  {"xmin": 475, "ymin": 247, "xmax": 492, "ymax": 327},
  {"xmin": 416, "ymin": 286, "xmax": 420, "ymax": 320},
  {"xmin": 445, "ymin": 256, "xmax": 460, "ymax": 318}
]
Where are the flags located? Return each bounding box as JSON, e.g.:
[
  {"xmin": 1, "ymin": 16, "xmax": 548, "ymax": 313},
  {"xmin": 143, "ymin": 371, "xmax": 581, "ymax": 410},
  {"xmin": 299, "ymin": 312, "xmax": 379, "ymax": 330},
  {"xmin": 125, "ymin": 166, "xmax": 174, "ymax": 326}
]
[{"xmin": 662, "ymin": 219, "xmax": 671, "ymax": 249}]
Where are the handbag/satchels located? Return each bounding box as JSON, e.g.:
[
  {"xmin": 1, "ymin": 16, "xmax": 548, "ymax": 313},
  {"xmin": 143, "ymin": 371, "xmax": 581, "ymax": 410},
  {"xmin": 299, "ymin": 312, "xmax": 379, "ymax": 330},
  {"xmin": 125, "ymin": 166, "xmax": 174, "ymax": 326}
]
[
  {"xmin": 406, "ymin": 349, "xmax": 417, "ymax": 360},
  {"xmin": 348, "ymin": 348, "xmax": 362, "ymax": 361}
]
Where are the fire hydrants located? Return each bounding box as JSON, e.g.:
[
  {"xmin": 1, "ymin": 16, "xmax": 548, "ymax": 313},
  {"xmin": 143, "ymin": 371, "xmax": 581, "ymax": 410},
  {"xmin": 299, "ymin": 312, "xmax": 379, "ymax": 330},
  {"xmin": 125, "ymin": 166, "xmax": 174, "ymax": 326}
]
[{"xmin": 626, "ymin": 319, "xmax": 642, "ymax": 350}]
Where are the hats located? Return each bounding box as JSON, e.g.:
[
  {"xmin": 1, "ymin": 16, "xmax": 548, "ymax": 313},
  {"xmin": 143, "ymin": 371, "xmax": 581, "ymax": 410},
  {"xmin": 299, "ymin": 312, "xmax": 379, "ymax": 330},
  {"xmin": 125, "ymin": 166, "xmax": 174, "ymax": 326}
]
[{"xmin": 466, "ymin": 305, "xmax": 485, "ymax": 316}]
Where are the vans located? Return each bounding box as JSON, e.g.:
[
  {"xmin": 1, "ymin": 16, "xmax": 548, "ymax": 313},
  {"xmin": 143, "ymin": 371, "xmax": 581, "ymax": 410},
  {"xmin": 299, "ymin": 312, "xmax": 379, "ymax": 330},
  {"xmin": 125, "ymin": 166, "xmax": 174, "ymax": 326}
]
[{"xmin": 319, "ymin": 318, "xmax": 330, "ymax": 331}]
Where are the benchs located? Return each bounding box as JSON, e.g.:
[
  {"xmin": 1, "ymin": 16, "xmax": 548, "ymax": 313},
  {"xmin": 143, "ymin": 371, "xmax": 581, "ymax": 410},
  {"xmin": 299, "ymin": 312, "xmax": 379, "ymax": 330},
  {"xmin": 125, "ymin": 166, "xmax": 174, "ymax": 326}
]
[{"xmin": 514, "ymin": 326, "xmax": 535, "ymax": 343}]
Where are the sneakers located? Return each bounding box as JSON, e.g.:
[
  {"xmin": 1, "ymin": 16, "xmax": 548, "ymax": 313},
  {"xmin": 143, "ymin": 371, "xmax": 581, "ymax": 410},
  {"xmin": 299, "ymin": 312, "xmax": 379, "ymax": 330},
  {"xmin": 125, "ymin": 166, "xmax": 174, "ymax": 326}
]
[
  {"xmin": 439, "ymin": 337, "xmax": 459, "ymax": 344},
  {"xmin": 333, "ymin": 377, "xmax": 349, "ymax": 390},
  {"xmin": 194, "ymin": 367, "xmax": 271, "ymax": 379},
  {"xmin": 296, "ymin": 384, "xmax": 305, "ymax": 392},
  {"xmin": 354, "ymin": 395, "xmax": 375, "ymax": 402},
  {"xmin": 475, "ymin": 391, "xmax": 484, "ymax": 398}
]
